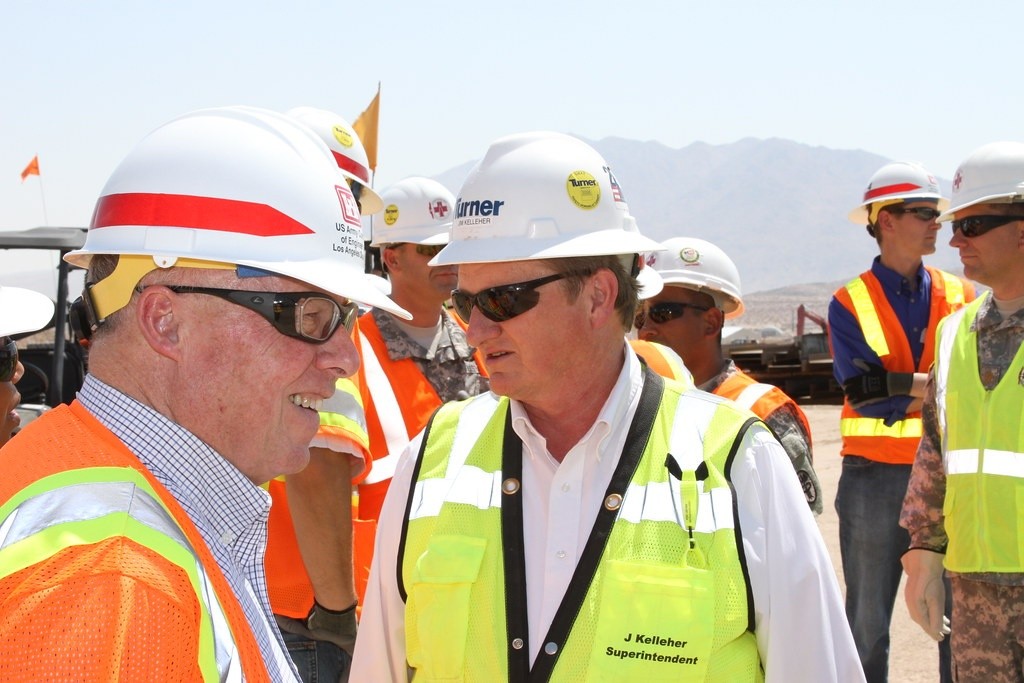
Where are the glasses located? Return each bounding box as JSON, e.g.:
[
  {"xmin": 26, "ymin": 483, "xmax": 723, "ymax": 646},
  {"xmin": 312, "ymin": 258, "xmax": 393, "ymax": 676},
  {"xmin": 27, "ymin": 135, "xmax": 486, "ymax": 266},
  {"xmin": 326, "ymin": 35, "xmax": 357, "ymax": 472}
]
[
  {"xmin": 450, "ymin": 266, "xmax": 590, "ymax": 324},
  {"xmin": 887, "ymin": 206, "xmax": 941, "ymax": 221},
  {"xmin": 0, "ymin": 340, "xmax": 17, "ymax": 382},
  {"xmin": 385, "ymin": 241, "xmax": 445, "ymax": 257},
  {"xmin": 136, "ymin": 285, "xmax": 359, "ymax": 344},
  {"xmin": 633, "ymin": 302, "xmax": 712, "ymax": 330},
  {"xmin": 951, "ymin": 214, "xmax": 1024, "ymax": 238}
]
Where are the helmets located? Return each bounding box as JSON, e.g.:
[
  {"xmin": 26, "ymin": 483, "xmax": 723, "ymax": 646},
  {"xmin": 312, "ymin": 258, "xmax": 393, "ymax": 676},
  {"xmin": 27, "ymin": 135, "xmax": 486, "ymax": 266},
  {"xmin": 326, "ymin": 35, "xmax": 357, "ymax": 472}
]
[
  {"xmin": 847, "ymin": 164, "xmax": 952, "ymax": 226},
  {"xmin": 643, "ymin": 236, "xmax": 744, "ymax": 319},
  {"xmin": 936, "ymin": 141, "xmax": 1024, "ymax": 225},
  {"xmin": 427, "ymin": 131, "xmax": 668, "ymax": 268},
  {"xmin": 286, "ymin": 107, "xmax": 385, "ymax": 216},
  {"xmin": 63, "ymin": 107, "xmax": 413, "ymax": 322},
  {"xmin": 0, "ymin": 285, "xmax": 55, "ymax": 337},
  {"xmin": 632, "ymin": 264, "xmax": 663, "ymax": 300},
  {"xmin": 369, "ymin": 177, "xmax": 456, "ymax": 248}
]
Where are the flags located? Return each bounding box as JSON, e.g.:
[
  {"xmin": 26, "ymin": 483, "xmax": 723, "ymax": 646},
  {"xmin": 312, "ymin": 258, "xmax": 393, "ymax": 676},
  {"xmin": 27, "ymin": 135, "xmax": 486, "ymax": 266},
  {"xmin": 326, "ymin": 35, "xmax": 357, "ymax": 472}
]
[
  {"xmin": 20, "ymin": 155, "xmax": 40, "ymax": 181},
  {"xmin": 351, "ymin": 93, "xmax": 380, "ymax": 172}
]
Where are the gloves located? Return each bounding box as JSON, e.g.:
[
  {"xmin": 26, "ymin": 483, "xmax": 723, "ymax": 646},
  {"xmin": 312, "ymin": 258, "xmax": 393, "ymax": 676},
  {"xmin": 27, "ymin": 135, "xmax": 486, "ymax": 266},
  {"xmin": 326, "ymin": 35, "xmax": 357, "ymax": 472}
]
[
  {"xmin": 900, "ymin": 548, "xmax": 951, "ymax": 642},
  {"xmin": 839, "ymin": 358, "xmax": 915, "ymax": 407},
  {"xmin": 274, "ymin": 594, "xmax": 357, "ymax": 657}
]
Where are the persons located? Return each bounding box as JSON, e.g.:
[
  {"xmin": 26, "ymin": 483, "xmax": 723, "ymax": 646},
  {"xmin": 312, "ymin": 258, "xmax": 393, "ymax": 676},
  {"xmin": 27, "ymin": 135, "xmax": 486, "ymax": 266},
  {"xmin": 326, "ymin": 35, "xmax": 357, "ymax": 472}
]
[
  {"xmin": 0, "ymin": 286, "xmax": 57, "ymax": 449},
  {"xmin": 824, "ymin": 161, "xmax": 978, "ymax": 683},
  {"xmin": 634, "ymin": 235, "xmax": 825, "ymax": 517},
  {"xmin": 0, "ymin": 102, "xmax": 415, "ymax": 683},
  {"xmin": 629, "ymin": 339, "xmax": 696, "ymax": 388},
  {"xmin": 346, "ymin": 133, "xmax": 868, "ymax": 683},
  {"xmin": 260, "ymin": 176, "xmax": 493, "ymax": 683},
  {"xmin": 899, "ymin": 142, "xmax": 1024, "ymax": 683}
]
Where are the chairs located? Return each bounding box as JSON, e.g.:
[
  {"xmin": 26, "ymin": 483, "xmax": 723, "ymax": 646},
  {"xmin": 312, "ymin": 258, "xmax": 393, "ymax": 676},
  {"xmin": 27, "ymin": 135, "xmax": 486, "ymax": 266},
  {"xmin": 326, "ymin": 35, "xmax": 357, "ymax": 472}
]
[{"xmin": 17, "ymin": 348, "xmax": 84, "ymax": 404}]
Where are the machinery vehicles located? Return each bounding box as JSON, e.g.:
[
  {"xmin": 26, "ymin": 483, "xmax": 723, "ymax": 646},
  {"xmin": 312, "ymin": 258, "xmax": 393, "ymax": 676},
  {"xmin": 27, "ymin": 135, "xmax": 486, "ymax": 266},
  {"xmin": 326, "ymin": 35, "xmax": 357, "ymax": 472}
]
[{"xmin": 719, "ymin": 301, "xmax": 845, "ymax": 400}]
